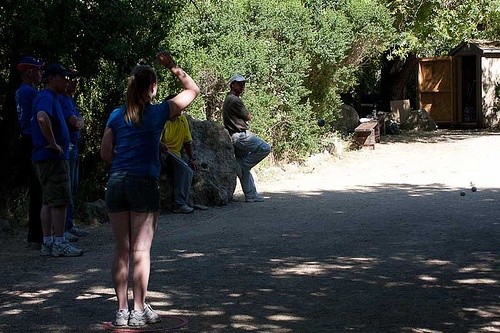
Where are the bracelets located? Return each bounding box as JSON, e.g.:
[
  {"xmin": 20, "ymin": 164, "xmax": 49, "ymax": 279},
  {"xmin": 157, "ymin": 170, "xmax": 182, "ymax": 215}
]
[
  {"xmin": 192, "ymin": 158, "xmax": 196, "ymax": 161},
  {"xmin": 166, "ymin": 61, "xmax": 174, "ymax": 69}
]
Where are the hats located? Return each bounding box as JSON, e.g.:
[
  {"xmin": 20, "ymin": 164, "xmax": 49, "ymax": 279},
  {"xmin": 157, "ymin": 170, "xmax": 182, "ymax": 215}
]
[
  {"xmin": 18, "ymin": 56, "xmax": 46, "ymax": 72},
  {"xmin": 67, "ymin": 72, "xmax": 77, "ymax": 78},
  {"xmin": 45, "ymin": 64, "xmax": 73, "ymax": 77},
  {"xmin": 230, "ymin": 75, "xmax": 249, "ymax": 85}
]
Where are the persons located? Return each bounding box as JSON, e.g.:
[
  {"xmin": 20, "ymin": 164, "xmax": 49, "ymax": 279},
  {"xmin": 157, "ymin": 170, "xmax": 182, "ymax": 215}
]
[
  {"xmin": 223, "ymin": 75, "xmax": 270, "ymax": 202},
  {"xmin": 57, "ymin": 71, "xmax": 90, "ymax": 241},
  {"xmin": 159, "ymin": 95, "xmax": 201, "ymax": 213},
  {"xmin": 31, "ymin": 65, "xmax": 84, "ymax": 256},
  {"xmin": 101, "ymin": 52, "xmax": 200, "ymax": 325},
  {"xmin": 14, "ymin": 57, "xmax": 43, "ymax": 249}
]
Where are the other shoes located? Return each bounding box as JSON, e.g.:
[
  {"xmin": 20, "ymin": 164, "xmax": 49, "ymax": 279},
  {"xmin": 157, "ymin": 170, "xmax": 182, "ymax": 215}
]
[
  {"xmin": 246, "ymin": 193, "xmax": 264, "ymax": 202},
  {"xmin": 23, "ymin": 242, "xmax": 42, "ymax": 257},
  {"xmin": 69, "ymin": 226, "xmax": 90, "ymax": 236},
  {"xmin": 64, "ymin": 232, "xmax": 79, "ymax": 241},
  {"xmin": 237, "ymin": 160, "xmax": 242, "ymax": 178}
]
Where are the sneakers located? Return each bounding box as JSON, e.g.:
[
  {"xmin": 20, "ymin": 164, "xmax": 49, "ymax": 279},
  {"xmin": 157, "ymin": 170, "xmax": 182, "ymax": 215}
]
[
  {"xmin": 113, "ymin": 307, "xmax": 133, "ymax": 325},
  {"xmin": 51, "ymin": 240, "xmax": 83, "ymax": 257},
  {"xmin": 128, "ymin": 303, "xmax": 165, "ymax": 326},
  {"xmin": 40, "ymin": 242, "xmax": 54, "ymax": 256},
  {"xmin": 172, "ymin": 204, "xmax": 194, "ymax": 213}
]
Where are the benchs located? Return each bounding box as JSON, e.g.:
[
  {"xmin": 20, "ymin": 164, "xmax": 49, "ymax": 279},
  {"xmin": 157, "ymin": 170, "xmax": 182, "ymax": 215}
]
[{"xmin": 355, "ymin": 116, "xmax": 383, "ymax": 149}]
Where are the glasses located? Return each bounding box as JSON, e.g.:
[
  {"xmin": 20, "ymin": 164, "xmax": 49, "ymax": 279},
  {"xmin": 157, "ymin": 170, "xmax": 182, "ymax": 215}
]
[
  {"xmin": 62, "ymin": 74, "xmax": 70, "ymax": 81},
  {"xmin": 72, "ymin": 78, "xmax": 80, "ymax": 81}
]
